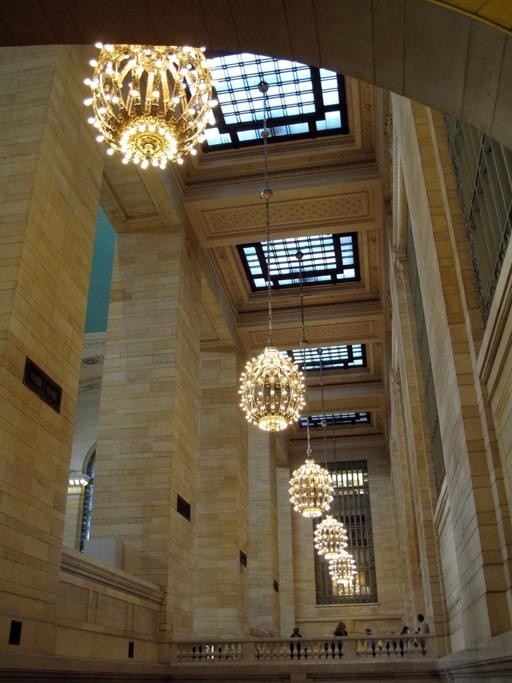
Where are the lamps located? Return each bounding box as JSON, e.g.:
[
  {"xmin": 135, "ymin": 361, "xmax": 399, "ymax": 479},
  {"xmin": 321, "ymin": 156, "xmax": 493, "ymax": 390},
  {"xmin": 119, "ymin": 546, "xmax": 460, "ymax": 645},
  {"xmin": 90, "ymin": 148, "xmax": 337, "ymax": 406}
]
[
  {"xmin": 289, "ymin": 251, "xmax": 334, "ymax": 519},
  {"xmin": 238, "ymin": 78, "xmax": 306, "ymax": 432},
  {"xmin": 313, "ymin": 347, "xmax": 350, "ymax": 560},
  {"xmin": 83, "ymin": 41, "xmax": 218, "ymax": 171},
  {"xmin": 329, "ymin": 554, "xmax": 358, "ymax": 586}
]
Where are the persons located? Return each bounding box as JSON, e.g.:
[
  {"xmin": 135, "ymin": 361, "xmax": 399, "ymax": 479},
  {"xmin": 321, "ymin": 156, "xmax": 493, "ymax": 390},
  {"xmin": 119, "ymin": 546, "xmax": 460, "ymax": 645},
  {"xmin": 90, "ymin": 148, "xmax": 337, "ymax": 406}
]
[
  {"xmin": 415, "ymin": 614, "xmax": 429, "ymax": 654},
  {"xmin": 334, "ymin": 623, "xmax": 347, "ymax": 657},
  {"xmin": 290, "ymin": 627, "xmax": 302, "ymax": 659},
  {"xmin": 366, "ymin": 625, "xmax": 410, "ymax": 656}
]
